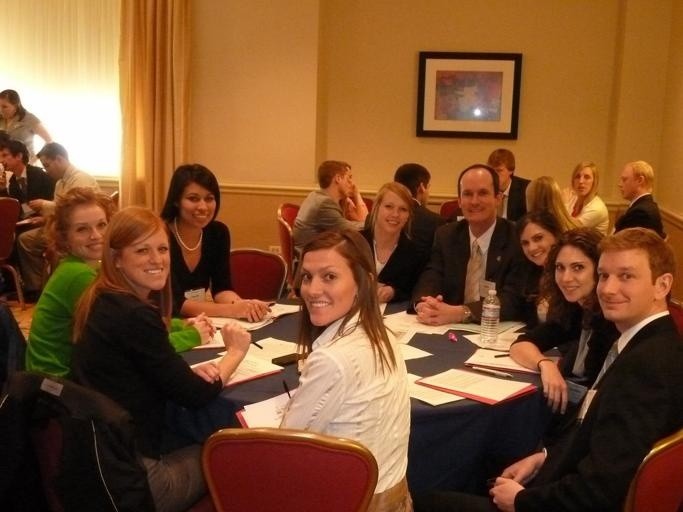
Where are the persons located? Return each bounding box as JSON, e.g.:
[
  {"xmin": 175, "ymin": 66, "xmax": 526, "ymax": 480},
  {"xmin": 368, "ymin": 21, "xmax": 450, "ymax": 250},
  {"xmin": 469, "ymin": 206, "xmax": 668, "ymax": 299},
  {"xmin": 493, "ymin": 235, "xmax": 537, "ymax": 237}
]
[
  {"xmin": 565, "ymin": 159, "xmax": 610, "ymax": 237},
  {"xmin": 488, "ymin": 148, "xmax": 531, "ymax": 222},
  {"xmin": 407, "ymin": 164, "xmax": 542, "ymax": 326},
  {"xmin": 415, "ymin": 226, "xmax": 683, "ymax": 512},
  {"xmin": 292, "ymin": 160, "xmax": 352, "ymax": 297},
  {"xmin": 340, "ymin": 173, "xmax": 370, "ymax": 222},
  {"xmin": 149, "ymin": 163, "xmax": 272, "ymax": 322},
  {"xmin": 612, "ymin": 159, "xmax": 663, "ymax": 239},
  {"xmin": 526, "ymin": 176, "xmax": 584, "ymax": 235},
  {"xmin": 70, "ymin": 205, "xmax": 252, "ymax": 512},
  {"xmin": 359, "ymin": 181, "xmax": 430, "ymax": 303},
  {"xmin": 0, "ymin": 140, "xmax": 52, "ymax": 220},
  {"xmin": 279, "ymin": 225, "xmax": 414, "ymax": 512},
  {"xmin": 515, "ymin": 209, "xmax": 564, "ymax": 330},
  {"xmin": 508, "ymin": 226, "xmax": 620, "ymax": 414},
  {"xmin": 23, "ymin": 186, "xmax": 217, "ymax": 379},
  {"xmin": 394, "ymin": 163, "xmax": 448, "ymax": 249},
  {"xmin": 0, "ymin": 89, "xmax": 54, "ymax": 166},
  {"xmin": 6, "ymin": 143, "xmax": 102, "ymax": 304}
]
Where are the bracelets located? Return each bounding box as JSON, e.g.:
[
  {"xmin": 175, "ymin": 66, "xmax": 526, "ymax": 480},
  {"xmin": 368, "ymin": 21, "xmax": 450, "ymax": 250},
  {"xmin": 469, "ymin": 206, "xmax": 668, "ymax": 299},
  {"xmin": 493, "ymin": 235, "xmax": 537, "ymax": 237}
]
[{"xmin": 538, "ymin": 359, "xmax": 554, "ymax": 372}]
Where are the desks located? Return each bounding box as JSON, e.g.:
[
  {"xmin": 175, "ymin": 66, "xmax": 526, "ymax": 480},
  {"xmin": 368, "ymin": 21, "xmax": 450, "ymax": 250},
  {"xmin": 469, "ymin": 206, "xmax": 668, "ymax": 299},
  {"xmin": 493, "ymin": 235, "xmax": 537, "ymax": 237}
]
[{"xmin": 169, "ymin": 302, "xmax": 565, "ymax": 512}]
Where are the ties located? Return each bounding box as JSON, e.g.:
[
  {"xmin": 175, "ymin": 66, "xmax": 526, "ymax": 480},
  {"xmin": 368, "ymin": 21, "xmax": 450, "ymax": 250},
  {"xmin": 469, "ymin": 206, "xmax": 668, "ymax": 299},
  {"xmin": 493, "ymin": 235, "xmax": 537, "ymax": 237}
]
[
  {"xmin": 463, "ymin": 241, "xmax": 484, "ymax": 305},
  {"xmin": 15, "ymin": 177, "xmax": 28, "ymax": 200},
  {"xmin": 497, "ymin": 193, "xmax": 502, "ymax": 218}
]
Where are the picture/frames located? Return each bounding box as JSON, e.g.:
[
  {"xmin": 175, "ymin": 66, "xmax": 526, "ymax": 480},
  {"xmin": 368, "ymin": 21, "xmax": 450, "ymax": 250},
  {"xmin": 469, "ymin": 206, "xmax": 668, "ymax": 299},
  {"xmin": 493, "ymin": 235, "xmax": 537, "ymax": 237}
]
[{"xmin": 416, "ymin": 49, "xmax": 522, "ymax": 138}]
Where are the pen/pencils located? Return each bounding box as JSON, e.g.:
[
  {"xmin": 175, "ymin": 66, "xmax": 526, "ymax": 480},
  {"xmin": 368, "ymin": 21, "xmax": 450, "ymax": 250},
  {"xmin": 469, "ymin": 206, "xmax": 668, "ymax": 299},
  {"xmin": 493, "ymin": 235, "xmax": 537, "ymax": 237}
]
[
  {"xmin": 251, "ymin": 340, "xmax": 263, "ymax": 349},
  {"xmin": 473, "ymin": 367, "xmax": 513, "ymax": 378},
  {"xmin": 281, "ymin": 379, "xmax": 291, "ymax": 399},
  {"xmin": 268, "ymin": 303, "xmax": 275, "ymax": 307},
  {"xmin": 494, "ymin": 353, "xmax": 510, "ymax": 358}
]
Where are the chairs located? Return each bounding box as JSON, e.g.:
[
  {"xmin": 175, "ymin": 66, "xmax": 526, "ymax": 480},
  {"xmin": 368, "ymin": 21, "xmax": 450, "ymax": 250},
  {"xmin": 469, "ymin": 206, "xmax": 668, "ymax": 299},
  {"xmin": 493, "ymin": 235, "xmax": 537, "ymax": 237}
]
[
  {"xmin": 201, "ymin": 428, "xmax": 378, "ymax": 511},
  {"xmin": 626, "ymin": 430, "xmax": 683, "ymax": 512},
  {"xmin": 228, "ymin": 249, "xmax": 287, "ymax": 301},
  {"xmin": 351, "ymin": 194, "xmax": 375, "ymax": 211},
  {"xmin": 0, "ymin": 368, "xmax": 135, "ymax": 512},
  {"xmin": 440, "ymin": 200, "xmax": 462, "ymax": 221},
  {"xmin": 0, "ymin": 197, "xmax": 28, "ymax": 310},
  {"xmin": 278, "ymin": 202, "xmax": 302, "ymax": 301}
]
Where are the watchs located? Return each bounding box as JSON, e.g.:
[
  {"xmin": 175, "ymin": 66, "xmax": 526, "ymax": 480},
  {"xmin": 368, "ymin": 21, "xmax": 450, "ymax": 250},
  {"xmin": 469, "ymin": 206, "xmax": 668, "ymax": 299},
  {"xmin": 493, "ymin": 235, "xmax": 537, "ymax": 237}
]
[{"xmin": 461, "ymin": 305, "xmax": 473, "ymax": 324}]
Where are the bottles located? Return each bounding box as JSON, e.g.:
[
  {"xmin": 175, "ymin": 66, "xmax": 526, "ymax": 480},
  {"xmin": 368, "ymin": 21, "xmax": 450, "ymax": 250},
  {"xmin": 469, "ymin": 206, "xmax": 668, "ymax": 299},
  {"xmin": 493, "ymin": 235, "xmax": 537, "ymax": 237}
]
[{"xmin": 481, "ymin": 291, "xmax": 499, "ymax": 346}]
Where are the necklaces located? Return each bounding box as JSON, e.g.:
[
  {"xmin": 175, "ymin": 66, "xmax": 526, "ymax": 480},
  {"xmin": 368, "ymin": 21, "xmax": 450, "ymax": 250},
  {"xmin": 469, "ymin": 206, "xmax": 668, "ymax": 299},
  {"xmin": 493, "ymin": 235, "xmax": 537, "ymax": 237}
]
[{"xmin": 174, "ymin": 216, "xmax": 202, "ymax": 252}]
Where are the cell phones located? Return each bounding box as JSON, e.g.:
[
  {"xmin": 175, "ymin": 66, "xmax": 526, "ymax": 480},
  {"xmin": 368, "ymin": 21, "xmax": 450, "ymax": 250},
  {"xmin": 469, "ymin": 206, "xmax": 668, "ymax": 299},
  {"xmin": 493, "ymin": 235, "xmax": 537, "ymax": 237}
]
[{"xmin": 272, "ymin": 353, "xmax": 301, "ymax": 365}]
